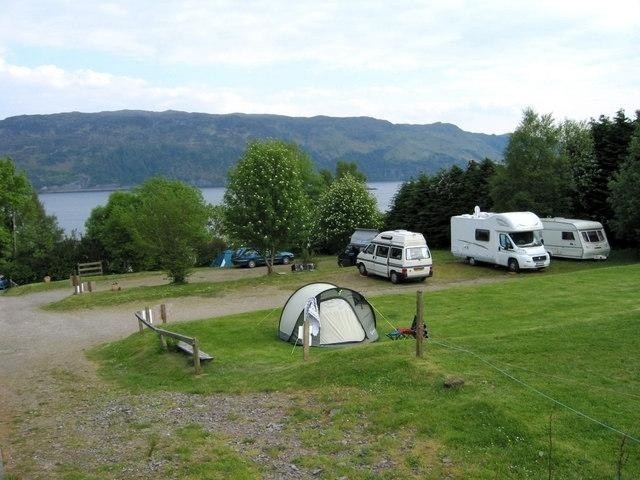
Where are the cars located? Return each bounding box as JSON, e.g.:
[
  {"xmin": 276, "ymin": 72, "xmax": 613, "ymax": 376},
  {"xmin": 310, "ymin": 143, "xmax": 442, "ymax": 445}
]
[
  {"xmin": 232, "ymin": 245, "xmax": 296, "ymax": 267},
  {"xmin": 344, "ymin": 229, "xmax": 380, "ymax": 264}
]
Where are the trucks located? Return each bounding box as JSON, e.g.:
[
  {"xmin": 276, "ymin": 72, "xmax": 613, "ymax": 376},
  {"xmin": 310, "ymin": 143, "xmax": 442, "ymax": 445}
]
[{"xmin": 450, "ymin": 210, "xmax": 552, "ymax": 274}]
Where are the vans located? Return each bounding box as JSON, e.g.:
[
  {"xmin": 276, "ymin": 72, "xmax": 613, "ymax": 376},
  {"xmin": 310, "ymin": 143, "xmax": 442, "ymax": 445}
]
[{"xmin": 355, "ymin": 229, "xmax": 434, "ymax": 284}]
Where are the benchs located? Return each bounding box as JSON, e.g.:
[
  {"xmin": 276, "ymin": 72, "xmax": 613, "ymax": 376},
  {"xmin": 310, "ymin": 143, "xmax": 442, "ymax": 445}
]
[{"xmin": 175, "ymin": 341, "xmax": 213, "ymax": 362}]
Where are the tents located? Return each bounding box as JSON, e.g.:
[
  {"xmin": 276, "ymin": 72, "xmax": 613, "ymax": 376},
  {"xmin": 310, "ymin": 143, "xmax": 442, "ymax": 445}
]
[
  {"xmin": 276, "ymin": 281, "xmax": 380, "ymax": 348},
  {"xmin": 209, "ymin": 249, "xmax": 238, "ymax": 269}
]
